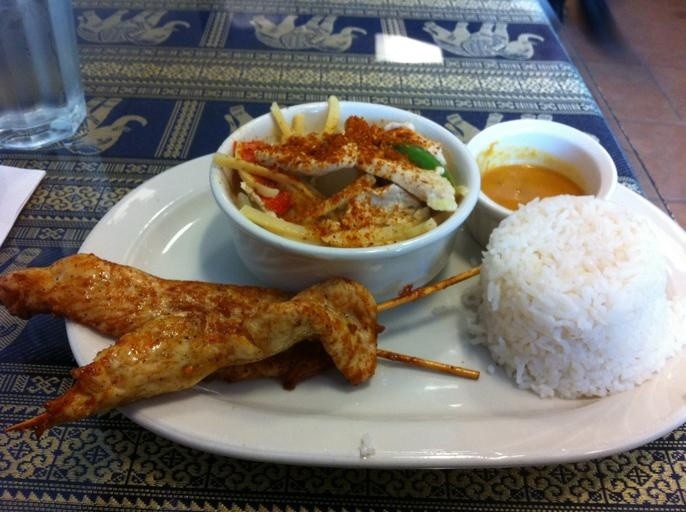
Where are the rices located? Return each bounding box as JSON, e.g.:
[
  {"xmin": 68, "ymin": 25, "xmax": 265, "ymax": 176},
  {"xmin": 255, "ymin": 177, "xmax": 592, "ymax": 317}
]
[{"xmin": 462, "ymin": 196, "xmax": 686, "ymax": 397}]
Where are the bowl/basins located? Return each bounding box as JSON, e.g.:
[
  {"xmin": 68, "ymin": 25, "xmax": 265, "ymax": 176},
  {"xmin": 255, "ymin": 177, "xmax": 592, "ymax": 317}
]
[
  {"xmin": 211, "ymin": 101, "xmax": 480, "ymax": 306},
  {"xmin": 466, "ymin": 119, "xmax": 616, "ymax": 252}
]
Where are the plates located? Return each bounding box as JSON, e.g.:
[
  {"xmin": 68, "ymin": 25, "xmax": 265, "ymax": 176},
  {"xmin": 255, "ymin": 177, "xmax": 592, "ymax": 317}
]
[{"xmin": 65, "ymin": 151, "xmax": 685, "ymax": 466}]
